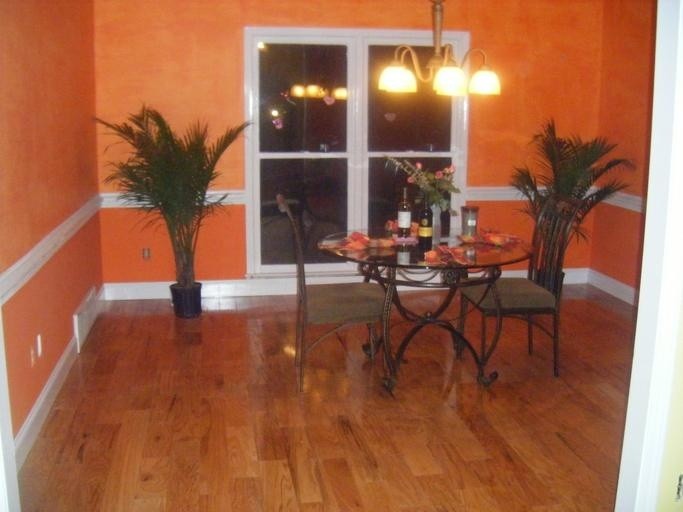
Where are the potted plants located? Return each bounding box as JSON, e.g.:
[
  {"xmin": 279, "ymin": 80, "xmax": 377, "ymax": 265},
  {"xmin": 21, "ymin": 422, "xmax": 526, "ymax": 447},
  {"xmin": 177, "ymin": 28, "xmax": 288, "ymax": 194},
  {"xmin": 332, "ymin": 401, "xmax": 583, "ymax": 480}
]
[
  {"xmin": 87, "ymin": 98, "xmax": 253, "ymax": 319},
  {"xmin": 512, "ymin": 112, "xmax": 634, "ymax": 303}
]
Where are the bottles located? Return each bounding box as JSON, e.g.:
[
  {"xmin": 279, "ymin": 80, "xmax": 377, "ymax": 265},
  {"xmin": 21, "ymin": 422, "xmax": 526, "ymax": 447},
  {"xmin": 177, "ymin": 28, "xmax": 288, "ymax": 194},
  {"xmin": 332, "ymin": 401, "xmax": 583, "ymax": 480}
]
[
  {"xmin": 419, "ymin": 192, "xmax": 432, "ymax": 250},
  {"xmin": 398, "ymin": 184, "xmax": 410, "ymax": 238},
  {"xmin": 440, "ymin": 191, "xmax": 449, "ymax": 237},
  {"xmin": 395, "ymin": 244, "xmax": 410, "ymax": 266}
]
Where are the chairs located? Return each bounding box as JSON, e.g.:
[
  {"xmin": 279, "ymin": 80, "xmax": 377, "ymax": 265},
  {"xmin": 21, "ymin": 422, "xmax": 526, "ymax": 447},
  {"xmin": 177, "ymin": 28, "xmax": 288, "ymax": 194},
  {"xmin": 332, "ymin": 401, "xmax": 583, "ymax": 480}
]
[
  {"xmin": 272, "ymin": 194, "xmax": 396, "ymax": 393},
  {"xmin": 453, "ymin": 197, "xmax": 576, "ymax": 382}
]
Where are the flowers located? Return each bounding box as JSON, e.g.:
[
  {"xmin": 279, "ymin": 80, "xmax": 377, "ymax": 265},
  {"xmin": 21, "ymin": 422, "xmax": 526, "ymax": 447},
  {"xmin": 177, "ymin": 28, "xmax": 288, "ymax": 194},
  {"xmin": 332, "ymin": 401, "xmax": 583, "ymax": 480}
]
[{"xmin": 387, "ymin": 156, "xmax": 463, "ymax": 215}]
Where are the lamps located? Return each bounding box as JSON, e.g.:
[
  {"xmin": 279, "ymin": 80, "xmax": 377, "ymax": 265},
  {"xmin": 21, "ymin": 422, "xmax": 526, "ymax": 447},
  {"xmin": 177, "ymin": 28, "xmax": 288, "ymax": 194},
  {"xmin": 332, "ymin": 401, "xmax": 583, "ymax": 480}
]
[{"xmin": 376, "ymin": 0, "xmax": 502, "ymax": 101}]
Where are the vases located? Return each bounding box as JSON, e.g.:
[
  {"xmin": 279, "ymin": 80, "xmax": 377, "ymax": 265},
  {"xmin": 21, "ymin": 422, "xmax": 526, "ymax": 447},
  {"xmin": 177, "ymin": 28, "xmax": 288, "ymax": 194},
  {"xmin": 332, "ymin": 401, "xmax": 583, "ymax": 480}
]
[{"xmin": 426, "ymin": 205, "xmax": 443, "ymax": 244}]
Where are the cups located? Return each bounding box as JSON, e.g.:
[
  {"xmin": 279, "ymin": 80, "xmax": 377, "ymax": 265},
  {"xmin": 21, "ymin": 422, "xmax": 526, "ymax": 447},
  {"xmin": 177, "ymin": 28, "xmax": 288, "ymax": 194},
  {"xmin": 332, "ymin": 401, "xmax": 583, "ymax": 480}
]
[
  {"xmin": 460, "ymin": 206, "xmax": 478, "ymax": 237},
  {"xmin": 461, "ymin": 244, "xmax": 476, "ymax": 267}
]
